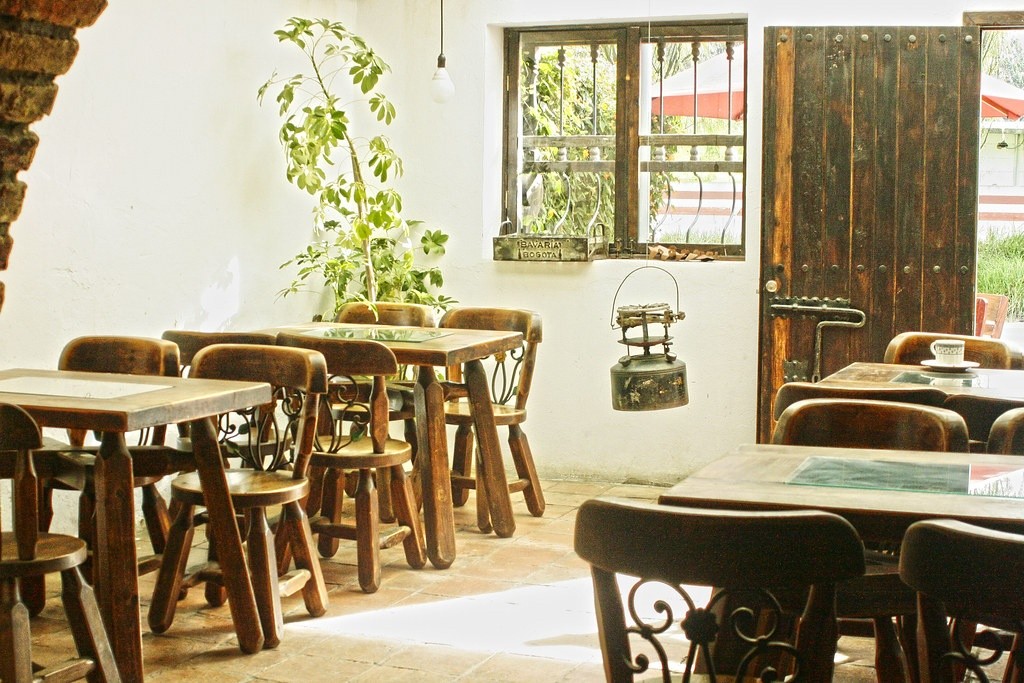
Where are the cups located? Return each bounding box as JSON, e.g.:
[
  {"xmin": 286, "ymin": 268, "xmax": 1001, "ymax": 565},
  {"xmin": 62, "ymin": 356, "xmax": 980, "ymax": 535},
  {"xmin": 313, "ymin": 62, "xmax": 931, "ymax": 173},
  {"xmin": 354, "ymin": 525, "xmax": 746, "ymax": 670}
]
[{"xmin": 930, "ymin": 340, "xmax": 964, "ymax": 363}]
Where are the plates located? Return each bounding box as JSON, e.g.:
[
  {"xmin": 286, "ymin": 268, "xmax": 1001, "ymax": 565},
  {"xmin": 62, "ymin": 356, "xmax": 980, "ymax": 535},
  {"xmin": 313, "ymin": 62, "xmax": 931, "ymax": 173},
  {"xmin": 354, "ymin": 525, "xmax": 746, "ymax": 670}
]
[{"xmin": 921, "ymin": 359, "xmax": 980, "ymax": 371}]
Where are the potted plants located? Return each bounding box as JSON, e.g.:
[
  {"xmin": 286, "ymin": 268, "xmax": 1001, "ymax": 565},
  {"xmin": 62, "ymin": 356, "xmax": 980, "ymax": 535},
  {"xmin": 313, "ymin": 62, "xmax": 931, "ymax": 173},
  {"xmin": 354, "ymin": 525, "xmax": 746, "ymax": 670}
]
[{"xmin": 253, "ymin": 13, "xmax": 453, "ymax": 382}]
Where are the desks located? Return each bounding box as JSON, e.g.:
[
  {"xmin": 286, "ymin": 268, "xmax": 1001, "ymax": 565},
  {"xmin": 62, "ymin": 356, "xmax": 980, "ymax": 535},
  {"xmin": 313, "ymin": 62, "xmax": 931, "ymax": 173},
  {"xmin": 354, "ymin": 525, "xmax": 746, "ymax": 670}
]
[
  {"xmin": 660, "ymin": 443, "xmax": 1022, "ymax": 672},
  {"xmin": 257, "ymin": 324, "xmax": 523, "ymax": 565},
  {"xmin": 0, "ymin": 369, "xmax": 272, "ymax": 683},
  {"xmin": 818, "ymin": 361, "xmax": 1024, "ymax": 407}
]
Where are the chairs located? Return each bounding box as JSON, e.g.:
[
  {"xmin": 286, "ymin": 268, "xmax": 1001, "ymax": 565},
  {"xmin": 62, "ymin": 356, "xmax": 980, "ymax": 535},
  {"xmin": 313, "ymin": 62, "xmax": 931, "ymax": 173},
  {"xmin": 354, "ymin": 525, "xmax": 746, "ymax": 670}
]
[
  {"xmin": 0, "ymin": 302, "xmax": 546, "ymax": 683},
  {"xmin": 575, "ymin": 331, "xmax": 1024, "ymax": 683}
]
